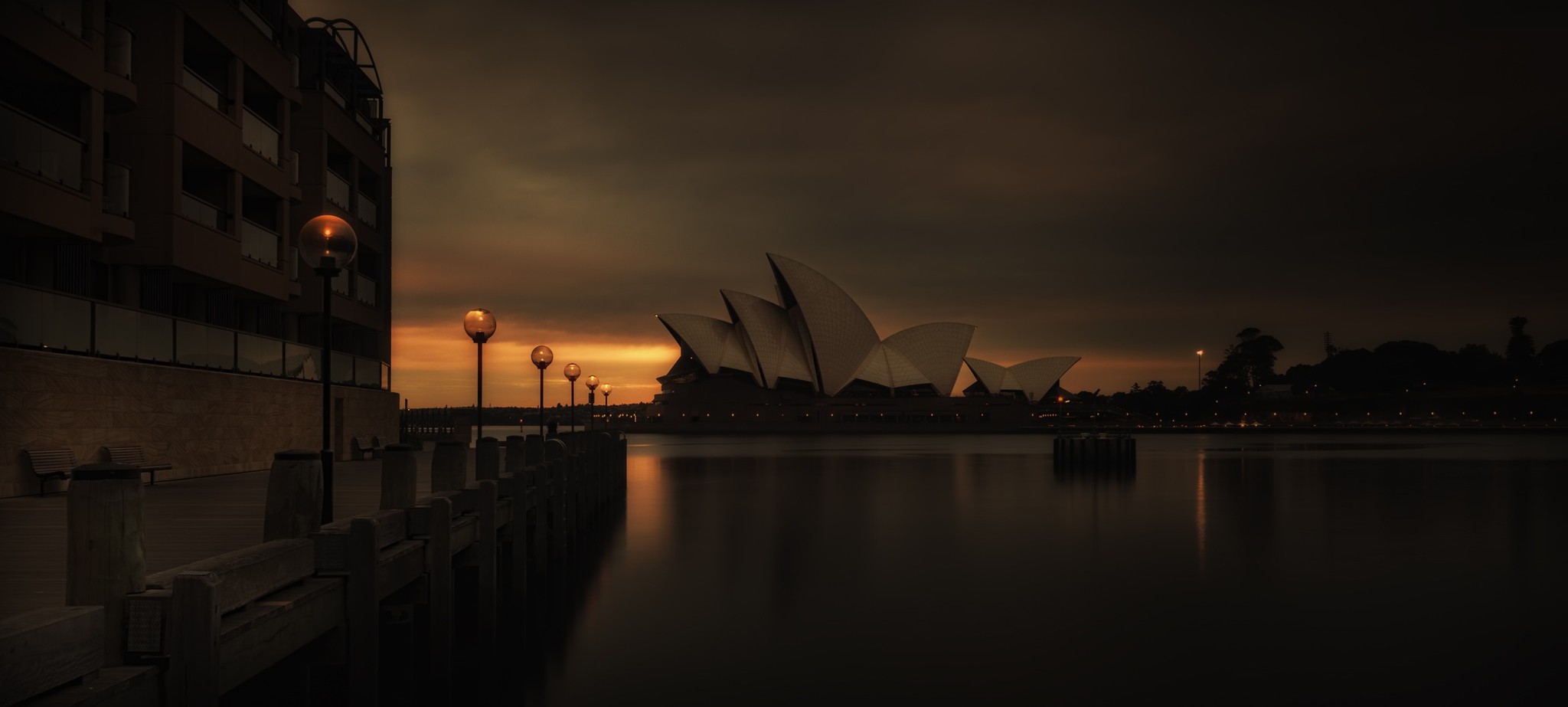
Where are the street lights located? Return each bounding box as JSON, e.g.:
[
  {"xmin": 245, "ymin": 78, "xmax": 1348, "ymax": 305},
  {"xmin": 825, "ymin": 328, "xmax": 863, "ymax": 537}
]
[
  {"xmin": 564, "ymin": 363, "xmax": 581, "ymax": 432},
  {"xmin": 1197, "ymin": 350, "xmax": 1204, "ymax": 391},
  {"xmin": 1058, "ymin": 396, "xmax": 1064, "ymax": 425},
  {"xmin": 300, "ymin": 214, "xmax": 358, "ymax": 529},
  {"xmin": 464, "ymin": 309, "xmax": 497, "ymax": 481},
  {"xmin": 601, "ymin": 384, "xmax": 613, "ymax": 432},
  {"xmin": 531, "ymin": 345, "xmax": 553, "ymax": 435},
  {"xmin": 586, "ymin": 375, "xmax": 599, "ymax": 431}
]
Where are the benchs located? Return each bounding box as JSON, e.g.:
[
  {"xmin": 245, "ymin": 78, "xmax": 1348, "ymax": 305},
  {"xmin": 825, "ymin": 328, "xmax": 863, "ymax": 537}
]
[
  {"xmin": 21, "ymin": 445, "xmax": 172, "ymax": 497},
  {"xmin": 353, "ymin": 436, "xmax": 388, "ymax": 462}
]
